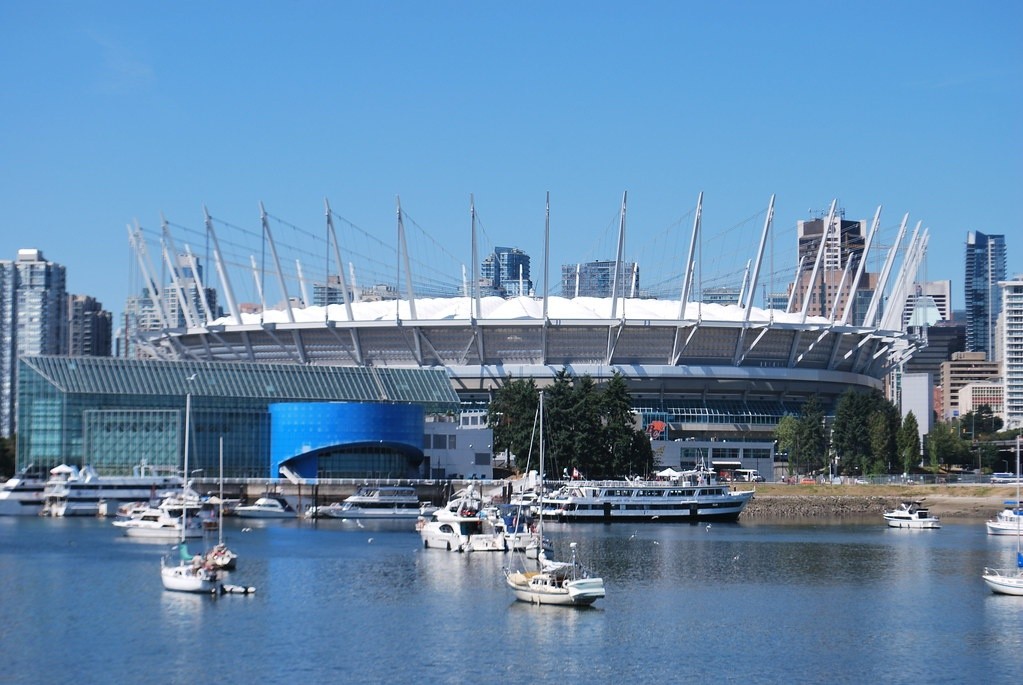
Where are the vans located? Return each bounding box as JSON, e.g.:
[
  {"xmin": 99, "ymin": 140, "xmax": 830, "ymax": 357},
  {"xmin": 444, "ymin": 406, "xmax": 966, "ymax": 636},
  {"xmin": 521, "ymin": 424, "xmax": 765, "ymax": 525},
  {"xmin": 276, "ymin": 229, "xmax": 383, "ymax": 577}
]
[{"xmin": 735, "ymin": 468, "xmax": 763, "ymax": 483}]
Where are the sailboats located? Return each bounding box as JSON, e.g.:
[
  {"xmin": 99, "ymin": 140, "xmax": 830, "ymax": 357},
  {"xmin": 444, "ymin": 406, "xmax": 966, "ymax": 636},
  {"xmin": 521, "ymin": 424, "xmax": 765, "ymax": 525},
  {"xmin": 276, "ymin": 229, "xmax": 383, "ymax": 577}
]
[
  {"xmin": 206, "ymin": 436, "xmax": 239, "ymax": 572},
  {"xmin": 525, "ymin": 437, "xmax": 556, "ymax": 562},
  {"xmin": 499, "ymin": 390, "xmax": 606, "ymax": 609},
  {"xmin": 981, "ymin": 434, "xmax": 1023, "ymax": 597},
  {"xmin": 157, "ymin": 391, "xmax": 232, "ymax": 597}
]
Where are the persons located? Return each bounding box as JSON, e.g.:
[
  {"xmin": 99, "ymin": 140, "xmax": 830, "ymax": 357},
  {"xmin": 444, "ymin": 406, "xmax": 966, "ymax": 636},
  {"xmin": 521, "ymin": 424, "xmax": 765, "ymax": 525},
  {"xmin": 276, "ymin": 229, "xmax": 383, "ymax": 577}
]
[
  {"xmin": 189, "ymin": 553, "xmax": 206, "ymax": 577},
  {"xmin": 697, "ymin": 474, "xmax": 702, "ymax": 486},
  {"xmin": 563, "ymin": 467, "xmax": 571, "ymax": 481},
  {"xmin": 656, "ymin": 477, "xmax": 660, "ymax": 481},
  {"xmin": 706, "ymin": 474, "xmax": 710, "ymax": 485},
  {"xmin": 647, "ymin": 476, "xmax": 651, "ymax": 481},
  {"xmin": 494, "ymin": 515, "xmax": 505, "ymax": 534},
  {"xmin": 538, "ymin": 549, "xmax": 547, "ymax": 575},
  {"xmin": 782, "ymin": 474, "xmax": 785, "ymax": 482}
]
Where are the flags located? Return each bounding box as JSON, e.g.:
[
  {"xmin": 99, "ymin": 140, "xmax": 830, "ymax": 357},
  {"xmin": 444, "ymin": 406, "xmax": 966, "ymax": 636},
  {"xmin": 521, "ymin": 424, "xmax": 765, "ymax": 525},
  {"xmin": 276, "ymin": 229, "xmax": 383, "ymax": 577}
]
[{"xmin": 573, "ymin": 467, "xmax": 578, "ymax": 477}]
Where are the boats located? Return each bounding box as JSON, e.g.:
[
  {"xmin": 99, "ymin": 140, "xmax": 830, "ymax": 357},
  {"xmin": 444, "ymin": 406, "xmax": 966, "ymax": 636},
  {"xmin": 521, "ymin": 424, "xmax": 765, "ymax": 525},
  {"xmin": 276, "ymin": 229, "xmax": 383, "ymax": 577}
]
[
  {"xmin": 985, "ymin": 501, "xmax": 1023, "ymax": 536},
  {"xmin": 110, "ymin": 475, "xmax": 245, "ymax": 544},
  {"xmin": 541, "ymin": 453, "xmax": 756, "ymax": 524},
  {"xmin": 883, "ymin": 496, "xmax": 943, "ymax": 529},
  {"xmin": 233, "ymin": 479, "xmax": 300, "ymax": 519},
  {"xmin": 320, "ymin": 484, "xmax": 421, "ymax": 520},
  {"xmin": 422, "ymin": 484, "xmax": 543, "ymax": 551},
  {"xmin": 0, "ymin": 459, "xmax": 201, "ymax": 519}
]
